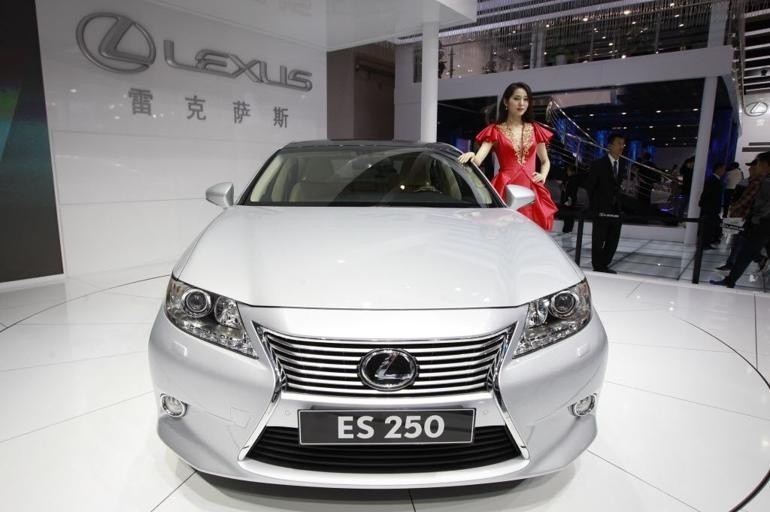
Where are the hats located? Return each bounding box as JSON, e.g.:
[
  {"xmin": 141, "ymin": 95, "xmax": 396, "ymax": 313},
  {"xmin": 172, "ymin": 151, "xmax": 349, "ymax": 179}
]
[{"xmin": 745, "ymin": 159, "xmax": 757, "ymax": 166}]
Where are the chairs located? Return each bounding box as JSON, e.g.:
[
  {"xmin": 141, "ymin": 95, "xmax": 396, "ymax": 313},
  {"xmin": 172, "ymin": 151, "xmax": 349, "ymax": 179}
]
[
  {"xmin": 398, "ymin": 156, "xmax": 426, "ymax": 187},
  {"xmin": 288, "ymin": 158, "xmax": 350, "ymax": 203}
]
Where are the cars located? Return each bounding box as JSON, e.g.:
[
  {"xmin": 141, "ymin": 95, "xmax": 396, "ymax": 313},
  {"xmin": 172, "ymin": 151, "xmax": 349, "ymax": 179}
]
[{"xmin": 146, "ymin": 137, "xmax": 610, "ymax": 494}]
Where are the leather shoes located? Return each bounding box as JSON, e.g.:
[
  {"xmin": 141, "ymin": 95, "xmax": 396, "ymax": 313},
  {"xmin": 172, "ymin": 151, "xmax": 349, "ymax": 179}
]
[
  {"xmin": 593, "ymin": 265, "xmax": 617, "ymax": 274},
  {"xmin": 716, "ymin": 263, "xmax": 732, "ymax": 271}
]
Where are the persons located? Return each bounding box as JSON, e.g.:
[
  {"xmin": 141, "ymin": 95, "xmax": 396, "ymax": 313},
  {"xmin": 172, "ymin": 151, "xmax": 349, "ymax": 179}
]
[
  {"xmin": 630, "ymin": 162, "xmax": 640, "ymax": 180},
  {"xmin": 715, "ymin": 157, "xmax": 770, "ymax": 274},
  {"xmin": 698, "ymin": 159, "xmax": 728, "ymax": 251},
  {"xmin": 457, "ymin": 81, "xmax": 560, "ymax": 239},
  {"xmin": 437, "ymin": 40, "xmax": 449, "ymax": 79},
  {"xmin": 632, "ymin": 151, "xmax": 657, "ymax": 224},
  {"xmin": 670, "ymin": 164, "xmax": 678, "ymax": 176},
  {"xmin": 723, "ymin": 161, "xmax": 743, "ymax": 220},
  {"xmin": 582, "ymin": 131, "xmax": 627, "ymax": 275},
  {"xmin": 559, "ymin": 164, "xmax": 581, "ymax": 236},
  {"xmin": 708, "ymin": 150, "xmax": 770, "ymax": 288}
]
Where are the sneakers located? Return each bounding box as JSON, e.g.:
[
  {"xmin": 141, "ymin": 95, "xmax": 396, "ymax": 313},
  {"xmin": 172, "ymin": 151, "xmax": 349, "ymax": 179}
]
[{"xmin": 710, "ymin": 279, "xmax": 736, "ymax": 288}]
